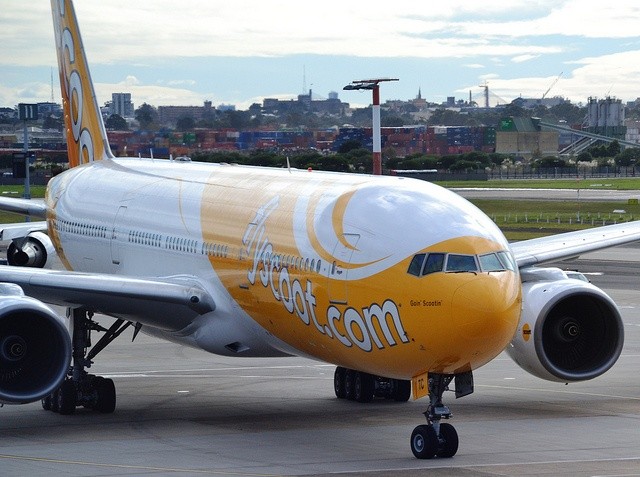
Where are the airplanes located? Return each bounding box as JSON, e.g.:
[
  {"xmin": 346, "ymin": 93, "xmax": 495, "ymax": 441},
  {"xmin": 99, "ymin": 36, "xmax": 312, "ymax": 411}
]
[{"xmin": 0, "ymin": 0, "xmax": 639, "ymax": 458}]
